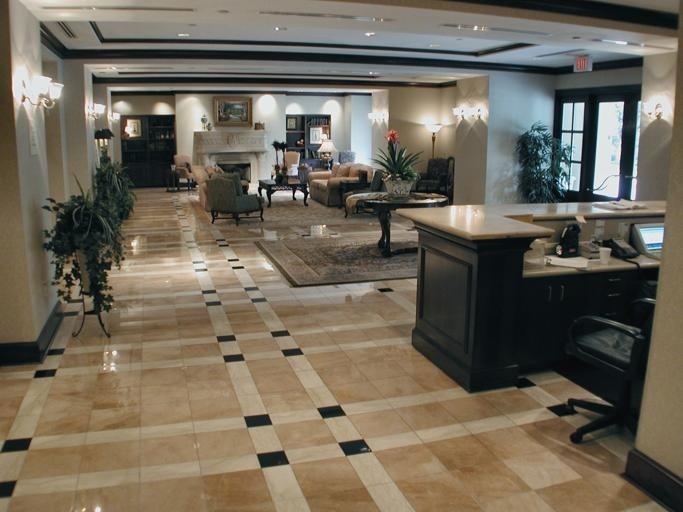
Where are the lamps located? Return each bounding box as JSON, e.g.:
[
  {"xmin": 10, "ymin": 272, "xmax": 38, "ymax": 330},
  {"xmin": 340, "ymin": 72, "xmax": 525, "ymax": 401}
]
[
  {"xmin": 317, "ymin": 142, "xmax": 338, "ymax": 171},
  {"xmin": 19, "ymin": 75, "xmax": 120, "ymax": 124},
  {"xmin": 367, "ymin": 104, "xmax": 484, "ymax": 159}
]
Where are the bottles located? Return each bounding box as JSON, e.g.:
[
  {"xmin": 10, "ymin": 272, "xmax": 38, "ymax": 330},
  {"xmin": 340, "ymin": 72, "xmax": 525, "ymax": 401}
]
[
  {"xmin": 206, "ymin": 122, "xmax": 213, "ymax": 130},
  {"xmin": 149, "ymin": 126, "xmax": 174, "ymax": 139},
  {"xmin": 201, "ymin": 112, "xmax": 208, "ymax": 130}
]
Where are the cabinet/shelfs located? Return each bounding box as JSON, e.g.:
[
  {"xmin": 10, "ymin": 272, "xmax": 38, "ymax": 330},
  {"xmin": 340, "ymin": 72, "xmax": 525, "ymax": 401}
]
[
  {"xmin": 516, "ymin": 266, "xmax": 659, "ymax": 362},
  {"xmin": 286, "ymin": 114, "xmax": 331, "ymax": 170}
]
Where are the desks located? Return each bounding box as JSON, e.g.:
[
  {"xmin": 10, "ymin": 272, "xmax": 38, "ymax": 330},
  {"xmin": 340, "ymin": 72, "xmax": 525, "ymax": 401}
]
[{"xmin": 347, "ymin": 193, "xmax": 449, "ymax": 258}]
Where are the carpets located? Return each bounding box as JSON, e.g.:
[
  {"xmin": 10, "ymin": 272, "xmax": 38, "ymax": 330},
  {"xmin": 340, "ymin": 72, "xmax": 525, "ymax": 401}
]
[{"xmin": 255, "ymin": 231, "xmax": 419, "ymax": 287}]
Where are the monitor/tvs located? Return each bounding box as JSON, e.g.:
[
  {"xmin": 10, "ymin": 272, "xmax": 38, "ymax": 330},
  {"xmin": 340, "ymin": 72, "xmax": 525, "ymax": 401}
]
[{"xmin": 630, "ymin": 221, "xmax": 664, "ymax": 252}]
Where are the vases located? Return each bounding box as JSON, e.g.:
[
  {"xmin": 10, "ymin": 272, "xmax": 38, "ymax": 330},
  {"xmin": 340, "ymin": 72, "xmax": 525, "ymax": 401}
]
[{"xmin": 382, "ymin": 178, "xmax": 415, "ymax": 198}]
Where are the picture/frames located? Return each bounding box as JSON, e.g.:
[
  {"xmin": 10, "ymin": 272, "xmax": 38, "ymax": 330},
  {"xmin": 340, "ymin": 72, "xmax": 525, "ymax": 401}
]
[
  {"xmin": 213, "ymin": 96, "xmax": 253, "ymax": 127},
  {"xmin": 286, "ymin": 117, "xmax": 297, "ymax": 130}
]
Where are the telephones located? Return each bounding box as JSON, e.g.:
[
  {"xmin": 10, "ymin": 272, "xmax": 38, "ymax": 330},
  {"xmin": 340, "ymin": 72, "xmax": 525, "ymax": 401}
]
[{"xmin": 602, "ymin": 237, "xmax": 640, "ymax": 258}]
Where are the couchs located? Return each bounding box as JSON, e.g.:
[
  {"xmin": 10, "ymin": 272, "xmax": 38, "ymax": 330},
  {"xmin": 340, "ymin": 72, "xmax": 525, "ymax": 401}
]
[{"xmin": 307, "ymin": 162, "xmax": 373, "ymax": 207}]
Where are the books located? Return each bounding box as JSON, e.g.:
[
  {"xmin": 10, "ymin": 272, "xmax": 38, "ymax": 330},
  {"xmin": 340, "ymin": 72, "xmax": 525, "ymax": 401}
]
[{"xmin": 593, "ymin": 197, "xmax": 646, "ymax": 210}]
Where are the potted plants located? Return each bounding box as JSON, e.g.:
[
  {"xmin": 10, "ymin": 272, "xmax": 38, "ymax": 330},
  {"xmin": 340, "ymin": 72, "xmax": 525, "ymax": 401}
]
[{"xmin": 40, "ymin": 152, "xmax": 138, "ymax": 314}]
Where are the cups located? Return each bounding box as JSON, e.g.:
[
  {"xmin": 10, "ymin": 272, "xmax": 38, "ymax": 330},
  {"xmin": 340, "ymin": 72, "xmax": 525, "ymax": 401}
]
[{"xmin": 599, "ymin": 246, "xmax": 611, "ymax": 264}]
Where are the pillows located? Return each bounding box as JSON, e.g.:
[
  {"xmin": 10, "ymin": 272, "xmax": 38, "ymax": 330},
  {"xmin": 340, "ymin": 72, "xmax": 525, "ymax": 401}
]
[
  {"xmin": 335, "ymin": 164, "xmax": 351, "ymax": 177},
  {"xmin": 348, "ymin": 166, "xmax": 361, "ymax": 177}
]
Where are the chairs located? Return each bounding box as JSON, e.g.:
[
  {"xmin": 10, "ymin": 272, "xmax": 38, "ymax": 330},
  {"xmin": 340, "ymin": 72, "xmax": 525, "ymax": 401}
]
[
  {"xmin": 416, "ymin": 157, "xmax": 455, "ymax": 205},
  {"xmin": 166, "ymin": 152, "xmax": 300, "ymax": 227},
  {"xmin": 561, "ymin": 297, "xmax": 655, "ymax": 442}
]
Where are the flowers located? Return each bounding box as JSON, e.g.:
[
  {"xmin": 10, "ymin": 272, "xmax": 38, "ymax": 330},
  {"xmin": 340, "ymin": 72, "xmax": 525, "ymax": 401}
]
[
  {"xmin": 278, "ymin": 142, "xmax": 289, "ymax": 166},
  {"xmin": 271, "ymin": 141, "xmax": 282, "ymax": 166},
  {"xmin": 370, "ymin": 129, "xmax": 426, "ymax": 184}
]
